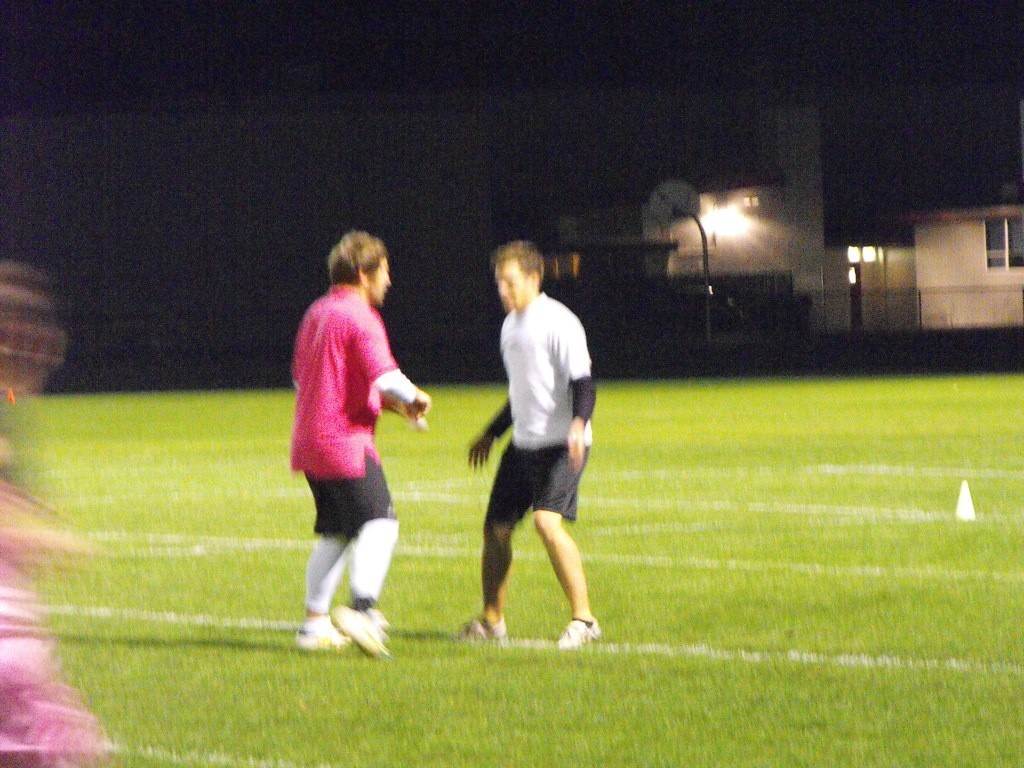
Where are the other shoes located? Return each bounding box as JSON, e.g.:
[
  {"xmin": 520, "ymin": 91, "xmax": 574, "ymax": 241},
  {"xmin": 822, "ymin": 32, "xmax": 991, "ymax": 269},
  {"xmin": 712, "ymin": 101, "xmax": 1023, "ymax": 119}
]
[
  {"xmin": 558, "ymin": 618, "xmax": 601, "ymax": 650},
  {"xmin": 454, "ymin": 619, "xmax": 507, "ymax": 644},
  {"xmin": 331, "ymin": 605, "xmax": 391, "ymax": 659},
  {"xmin": 295, "ymin": 626, "xmax": 351, "ymax": 649}
]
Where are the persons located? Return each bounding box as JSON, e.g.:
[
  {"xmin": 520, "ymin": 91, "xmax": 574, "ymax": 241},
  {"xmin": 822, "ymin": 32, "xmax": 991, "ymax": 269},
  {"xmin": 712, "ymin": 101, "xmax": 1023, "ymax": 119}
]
[
  {"xmin": 290, "ymin": 233, "xmax": 432, "ymax": 659},
  {"xmin": 460, "ymin": 243, "xmax": 602, "ymax": 647},
  {"xmin": 1, "ymin": 260, "xmax": 105, "ymax": 768}
]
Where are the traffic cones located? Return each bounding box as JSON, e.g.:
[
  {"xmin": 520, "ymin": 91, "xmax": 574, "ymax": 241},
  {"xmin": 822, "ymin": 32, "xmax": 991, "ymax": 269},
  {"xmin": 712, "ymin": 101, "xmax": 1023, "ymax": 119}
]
[{"xmin": 955, "ymin": 479, "xmax": 977, "ymax": 522}]
